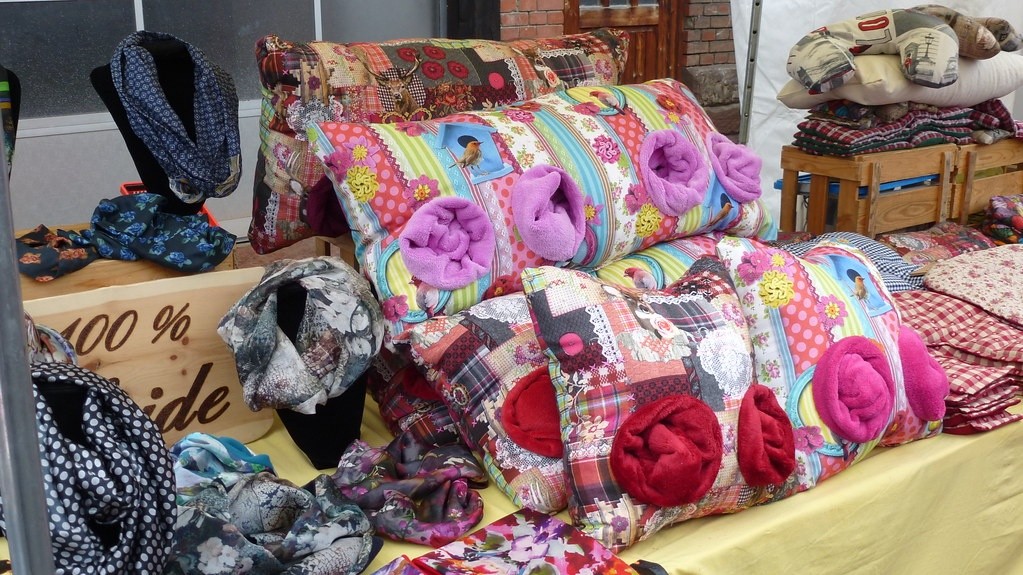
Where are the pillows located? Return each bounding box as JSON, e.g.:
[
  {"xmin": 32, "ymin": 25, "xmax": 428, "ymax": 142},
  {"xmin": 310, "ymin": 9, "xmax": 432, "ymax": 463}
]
[
  {"xmin": 776, "ymin": 49, "xmax": 1023, "ymax": 108},
  {"xmin": 247, "ymin": 25, "xmax": 633, "ymax": 256},
  {"xmin": 717, "ymin": 237, "xmax": 950, "ymax": 485},
  {"xmin": 309, "ymin": 76, "xmax": 780, "ymax": 352},
  {"xmin": 519, "ymin": 259, "xmax": 808, "ymax": 554}
]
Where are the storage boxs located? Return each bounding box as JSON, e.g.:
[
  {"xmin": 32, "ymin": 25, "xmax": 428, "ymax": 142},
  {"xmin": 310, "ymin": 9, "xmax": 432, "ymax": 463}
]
[{"xmin": 773, "ymin": 175, "xmax": 936, "ymax": 225}]
